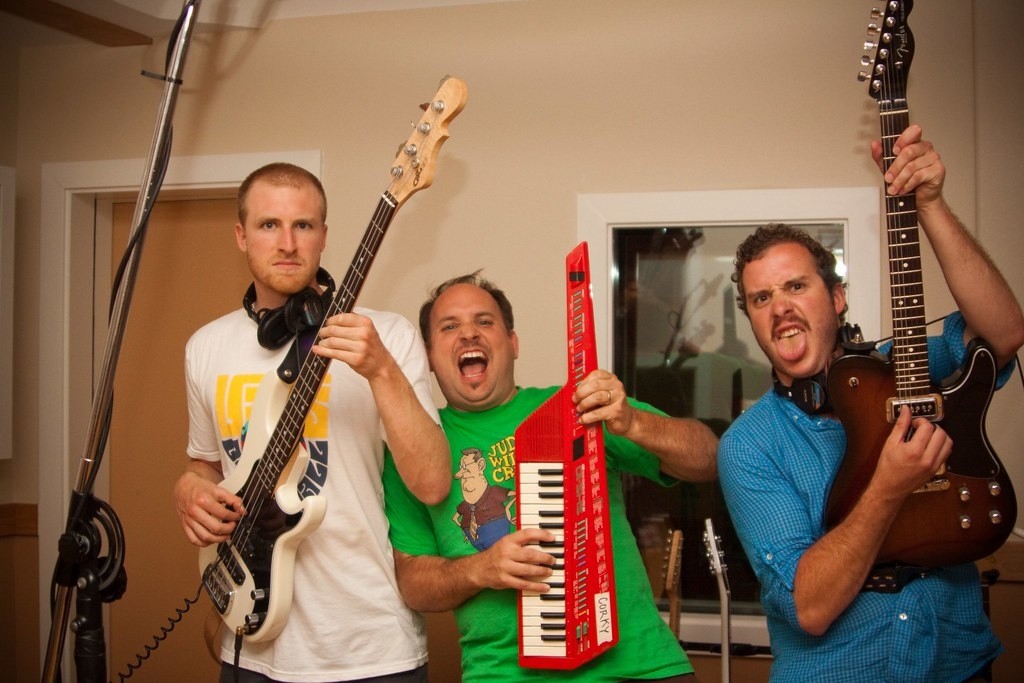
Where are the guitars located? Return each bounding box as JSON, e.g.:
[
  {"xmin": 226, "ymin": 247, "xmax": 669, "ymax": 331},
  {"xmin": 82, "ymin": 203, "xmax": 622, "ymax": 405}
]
[
  {"xmin": 820, "ymin": 0, "xmax": 1019, "ymax": 565},
  {"xmin": 703, "ymin": 518, "xmax": 733, "ymax": 683},
  {"xmin": 671, "ymin": 318, "xmax": 716, "ymax": 371},
  {"xmin": 716, "ymin": 284, "xmax": 750, "ymax": 359},
  {"xmin": 662, "ymin": 528, "xmax": 684, "ymax": 638},
  {"xmin": 199, "ymin": 76, "xmax": 467, "ymax": 645}
]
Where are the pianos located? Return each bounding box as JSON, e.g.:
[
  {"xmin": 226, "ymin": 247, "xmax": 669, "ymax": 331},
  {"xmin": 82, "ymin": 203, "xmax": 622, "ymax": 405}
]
[{"xmin": 514, "ymin": 239, "xmax": 620, "ymax": 671}]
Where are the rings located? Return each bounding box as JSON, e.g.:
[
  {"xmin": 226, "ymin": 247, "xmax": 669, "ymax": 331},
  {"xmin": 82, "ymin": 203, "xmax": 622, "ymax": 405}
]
[{"xmin": 606, "ymin": 390, "xmax": 611, "ymax": 403}]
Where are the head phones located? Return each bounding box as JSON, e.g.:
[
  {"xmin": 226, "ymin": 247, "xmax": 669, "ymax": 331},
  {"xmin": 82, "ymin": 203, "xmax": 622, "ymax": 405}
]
[
  {"xmin": 770, "ymin": 323, "xmax": 862, "ymax": 417},
  {"xmin": 243, "ymin": 268, "xmax": 335, "ymax": 350}
]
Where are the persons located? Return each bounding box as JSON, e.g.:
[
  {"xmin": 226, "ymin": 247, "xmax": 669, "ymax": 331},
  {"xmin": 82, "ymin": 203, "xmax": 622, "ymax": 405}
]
[
  {"xmin": 383, "ymin": 274, "xmax": 722, "ymax": 683},
  {"xmin": 173, "ymin": 162, "xmax": 452, "ymax": 683},
  {"xmin": 717, "ymin": 125, "xmax": 1024, "ymax": 683}
]
